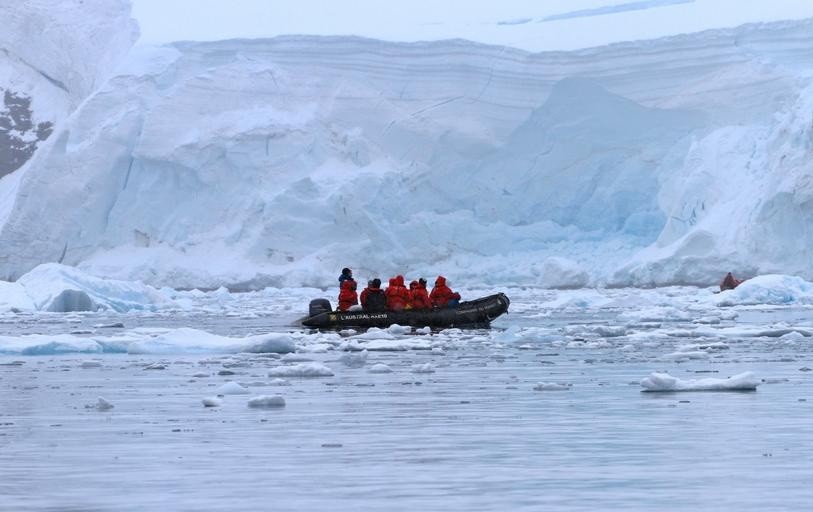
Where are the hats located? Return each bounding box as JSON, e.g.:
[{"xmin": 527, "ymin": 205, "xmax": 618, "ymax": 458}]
[
  {"xmin": 435, "ymin": 277, "xmax": 446, "ymax": 287},
  {"xmin": 409, "ymin": 278, "xmax": 427, "ymax": 289},
  {"xmin": 368, "ymin": 279, "xmax": 381, "ymax": 285},
  {"xmin": 342, "ymin": 268, "xmax": 351, "ymax": 274},
  {"xmin": 389, "ymin": 276, "xmax": 404, "ymax": 286}
]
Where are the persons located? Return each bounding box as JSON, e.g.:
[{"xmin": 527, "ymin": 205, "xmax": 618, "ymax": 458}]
[
  {"xmin": 384, "ymin": 278, "xmax": 411, "ymax": 311},
  {"xmin": 410, "ymin": 278, "xmax": 433, "ymax": 310},
  {"xmin": 407, "ymin": 280, "xmax": 424, "ymax": 309},
  {"xmin": 427, "ymin": 275, "xmax": 462, "ymax": 310},
  {"xmin": 395, "ymin": 273, "xmax": 406, "ymax": 288},
  {"xmin": 366, "ymin": 278, "xmax": 373, "ymax": 289},
  {"xmin": 720, "ymin": 270, "xmax": 738, "ymax": 290},
  {"xmin": 335, "ymin": 267, "xmax": 362, "ymax": 312},
  {"xmin": 358, "ymin": 278, "xmax": 388, "ymax": 313}
]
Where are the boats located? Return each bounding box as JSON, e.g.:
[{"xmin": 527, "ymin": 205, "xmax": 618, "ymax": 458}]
[
  {"xmin": 717, "ymin": 278, "xmax": 744, "ymax": 292},
  {"xmin": 299, "ymin": 290, "xmax": 513, "ymax": 332}
]
